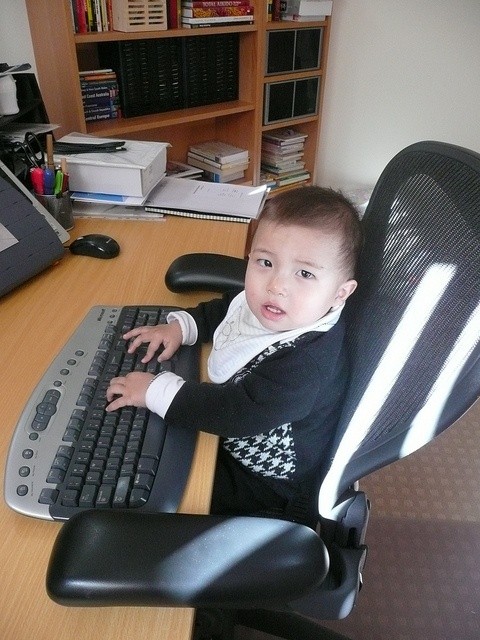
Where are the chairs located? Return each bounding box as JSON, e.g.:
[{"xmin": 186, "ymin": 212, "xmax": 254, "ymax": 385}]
[{"xmin": 46, "ymin": 139, "xmax": 479, "ymax": 640}]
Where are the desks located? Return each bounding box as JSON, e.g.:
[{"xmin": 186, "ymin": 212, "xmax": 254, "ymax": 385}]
[{"xmin": 1, "ymin": 213, "xmax": 261, "ymax": 639}]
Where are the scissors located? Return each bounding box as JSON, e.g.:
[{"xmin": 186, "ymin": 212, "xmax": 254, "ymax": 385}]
[{"xmin": 12, "ymin": 132, "xmax": 46, "ymax": 171}]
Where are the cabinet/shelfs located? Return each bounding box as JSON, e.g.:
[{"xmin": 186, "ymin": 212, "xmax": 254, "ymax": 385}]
[
  {"xmin": 258, "ymin": 19, "xmax": 332, "ymax": 197},
  {"xmin": 25, "ymin": 1, "xmax": 263, "ymax": 200}
]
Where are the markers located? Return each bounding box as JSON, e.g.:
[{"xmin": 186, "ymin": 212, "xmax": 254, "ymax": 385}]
[
  {"xmin": 30, "ymin": 167, "xmax": 44, "ymax": 195},
  {"xmin": 43, "ymin": 169, "xmax": 55, "ymax": 196}
]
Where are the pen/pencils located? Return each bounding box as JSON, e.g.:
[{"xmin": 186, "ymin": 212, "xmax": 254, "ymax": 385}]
[
  {"xmin": 62, "ymin": 173, "xmax": 69, "ymax": 191},
  {"xmin": 52, "ymin": 170, "xmax": 63, "ymax": 195},
  {"xmin": 47, "ymin": 135, "xmax": 54, "ymax": 174}
]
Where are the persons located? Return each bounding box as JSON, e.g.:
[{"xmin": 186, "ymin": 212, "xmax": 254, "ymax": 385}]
[{"xmin": 105, "ymin": 186, "xmax": 365, "ymax": 512}]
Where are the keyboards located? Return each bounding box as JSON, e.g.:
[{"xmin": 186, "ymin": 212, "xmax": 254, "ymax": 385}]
[{"xmin": 2, "ymin": 304, "xmax": 200, "ymax": 522}]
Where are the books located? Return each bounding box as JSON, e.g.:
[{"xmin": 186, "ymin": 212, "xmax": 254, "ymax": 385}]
[
  {"xmin": 182, "ymin": 21, "xmax": 254, "ymax": 29},
  {"xmin": 260, "ymin": 128, "xmax": 311, "ymax": 188},
  {"xmin": 182, "ymin": 7, "xmax": 255, "ymax": 15},
  {"xmin": 273, "ymin": 15, "xmax": 326, "ymax": 22},
  {"xmin": 73, "ymin": 0, "xmax": 112, "ymax": 32},
  {"xmin": 182, "ymin": 16, "xmax": 255, "ymax": 24},
  {"xmin": 77, "ymin": 69, "xmax": 119, "ymax": 121},
  {"xmin": 169, "ymin": 141, "xmax": 248, "ymax": 183},
  {"xmin": 31, "ymin": 132, "xmax": 169, "ymax": 196},
  {"xmin": 180, "ymin": 1, "xmax": 249, "ymax": 7},
  {"xmin": 271, "ymin": 0, "xmax": 332, "ymax": 16}
]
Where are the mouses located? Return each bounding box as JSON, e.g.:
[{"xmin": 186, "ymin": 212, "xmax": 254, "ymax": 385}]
[{"xmin": 67, "ymin": 233, "xmax": 122, "ymax": 260}]
